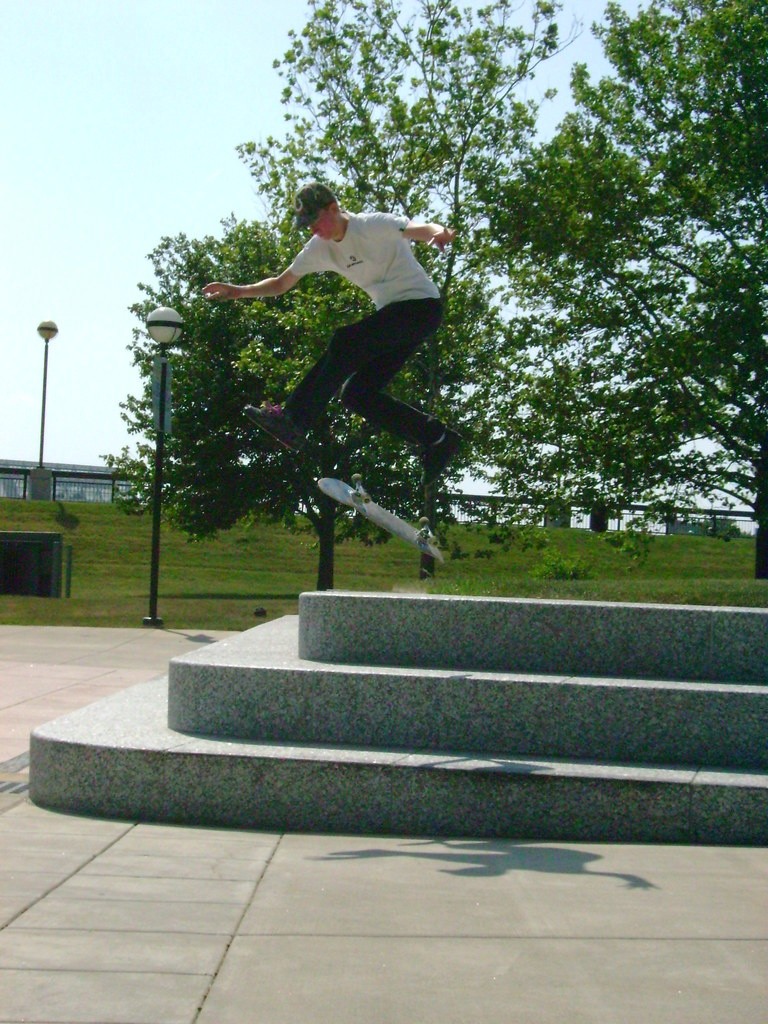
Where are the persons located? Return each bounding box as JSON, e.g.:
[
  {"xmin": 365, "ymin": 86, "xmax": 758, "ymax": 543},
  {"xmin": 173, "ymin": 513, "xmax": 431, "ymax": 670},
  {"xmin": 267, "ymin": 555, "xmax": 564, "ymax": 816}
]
[{"xmin": 202, "ymin": 183, "xmax": 462, "ymax": 484}]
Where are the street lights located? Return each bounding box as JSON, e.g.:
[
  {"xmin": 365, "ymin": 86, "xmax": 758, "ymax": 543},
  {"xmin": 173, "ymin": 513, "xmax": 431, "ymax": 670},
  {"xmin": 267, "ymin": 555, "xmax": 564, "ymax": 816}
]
[
  {"xmin": 27, "ymin": 320, "xmax": 59, "ymax": 501},
  {"xmin": 142, "ymin": 306, "xmax": 186, "ymax": 625}
]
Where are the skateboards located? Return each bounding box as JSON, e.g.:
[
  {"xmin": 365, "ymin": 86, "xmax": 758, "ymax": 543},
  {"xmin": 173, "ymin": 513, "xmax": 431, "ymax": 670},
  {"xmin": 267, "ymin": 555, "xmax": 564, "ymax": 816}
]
[{"xmin": 316, "ymin": 472, "xmax": 446, "ymax": 565}]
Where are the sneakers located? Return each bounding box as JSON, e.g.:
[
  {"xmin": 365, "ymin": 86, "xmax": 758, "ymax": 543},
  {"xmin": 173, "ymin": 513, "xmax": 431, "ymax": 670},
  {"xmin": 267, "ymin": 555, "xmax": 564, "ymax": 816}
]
[
  {"xmin": 242, "ymin": 402, "xmax": 308, "ymax": 452},
  {"xmin": 416, "ymin": 426, "xmax": 464, "ymax": 485}
]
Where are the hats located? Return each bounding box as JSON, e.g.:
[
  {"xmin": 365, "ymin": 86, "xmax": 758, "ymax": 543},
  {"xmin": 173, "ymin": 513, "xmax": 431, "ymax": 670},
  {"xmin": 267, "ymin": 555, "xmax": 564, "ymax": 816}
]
[{"xmin": 290, "ymin": 182, "xmax": 337, "ymax": 229}]
